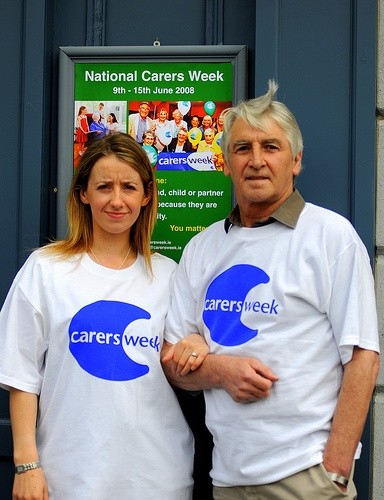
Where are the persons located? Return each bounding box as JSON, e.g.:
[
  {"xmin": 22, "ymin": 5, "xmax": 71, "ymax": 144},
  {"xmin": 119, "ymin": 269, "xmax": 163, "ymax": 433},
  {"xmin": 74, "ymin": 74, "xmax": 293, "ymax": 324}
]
[
  {"xmin": 74, "ymin": 100, "xmax": 231, "ymax": 172},
  {"xmin": 0, "ymin": 133, "xmax": 211, "ymax": 500},
  {"xmin": 157, "ymin": 78, "xmax": 380, "ymax": 500}
]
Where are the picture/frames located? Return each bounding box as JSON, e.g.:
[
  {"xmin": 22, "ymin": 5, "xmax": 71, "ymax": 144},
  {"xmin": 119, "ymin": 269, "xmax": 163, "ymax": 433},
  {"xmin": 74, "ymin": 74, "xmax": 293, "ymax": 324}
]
[{"xmin": 59, "ymin": 45, "xmax": 247, "ymax": 264}]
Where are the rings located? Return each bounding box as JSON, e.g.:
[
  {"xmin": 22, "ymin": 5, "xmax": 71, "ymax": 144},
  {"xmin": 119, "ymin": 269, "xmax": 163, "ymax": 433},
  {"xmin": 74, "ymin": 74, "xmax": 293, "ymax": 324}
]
[{"xmin": 191, "ymin": 353, "xmax": 198, "ymax": 358}]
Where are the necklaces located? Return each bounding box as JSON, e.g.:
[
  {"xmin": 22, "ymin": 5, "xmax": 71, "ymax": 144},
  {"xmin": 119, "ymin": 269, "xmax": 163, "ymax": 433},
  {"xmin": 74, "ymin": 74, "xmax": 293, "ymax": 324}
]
[{"xmin": 86, "ymin": 237, "xmax": 135, "ymax": 270}]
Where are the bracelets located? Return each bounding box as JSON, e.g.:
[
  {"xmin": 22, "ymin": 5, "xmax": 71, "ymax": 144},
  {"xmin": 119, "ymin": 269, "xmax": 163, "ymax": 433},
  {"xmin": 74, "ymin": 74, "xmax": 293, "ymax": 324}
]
[{"xmin": 326, "ymin": 471, "xmax": 348, "ymax": 490}]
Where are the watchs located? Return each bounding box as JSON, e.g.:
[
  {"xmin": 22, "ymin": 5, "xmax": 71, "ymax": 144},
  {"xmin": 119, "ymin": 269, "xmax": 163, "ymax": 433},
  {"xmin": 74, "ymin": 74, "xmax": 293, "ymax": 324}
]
[{"xmin": 14, "ymin": 459, "xmax": 41, "ymax": 474}]
